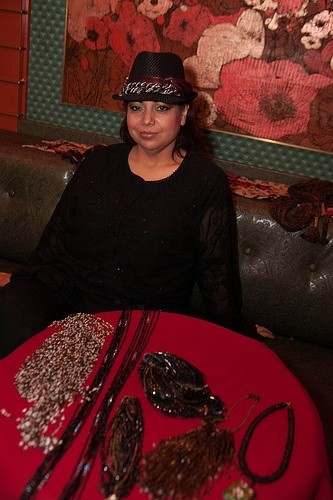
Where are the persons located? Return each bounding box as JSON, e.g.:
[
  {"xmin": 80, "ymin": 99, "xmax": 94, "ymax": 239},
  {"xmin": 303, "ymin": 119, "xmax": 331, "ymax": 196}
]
[{"xmin": 0, "ymin": 51, "xmax": 274, "ymax": 360}]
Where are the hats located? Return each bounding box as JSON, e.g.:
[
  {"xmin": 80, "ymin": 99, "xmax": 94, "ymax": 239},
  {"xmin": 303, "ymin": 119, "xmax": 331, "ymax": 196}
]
[{"xmin": 112, "ymin": 50, "xmax": 198, "ymax": 105}]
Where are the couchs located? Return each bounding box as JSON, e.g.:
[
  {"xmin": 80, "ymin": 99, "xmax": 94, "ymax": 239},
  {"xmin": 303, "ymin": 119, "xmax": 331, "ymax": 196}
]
[{"xmin": 0, "ymin": 145, "xmax": 332, "ymax": 442}]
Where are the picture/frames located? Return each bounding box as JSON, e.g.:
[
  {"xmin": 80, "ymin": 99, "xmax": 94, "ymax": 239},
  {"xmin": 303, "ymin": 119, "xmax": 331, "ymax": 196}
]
[{"xmin": 25, "ymin": 0, "xmax": 332, "ymax": 181}]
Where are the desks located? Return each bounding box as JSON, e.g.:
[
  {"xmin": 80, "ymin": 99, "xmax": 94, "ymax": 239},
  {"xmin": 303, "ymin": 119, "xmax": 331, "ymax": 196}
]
[{"xmin": 0, "ymin": 310, "xmax": 333, "ymax": 500}]
